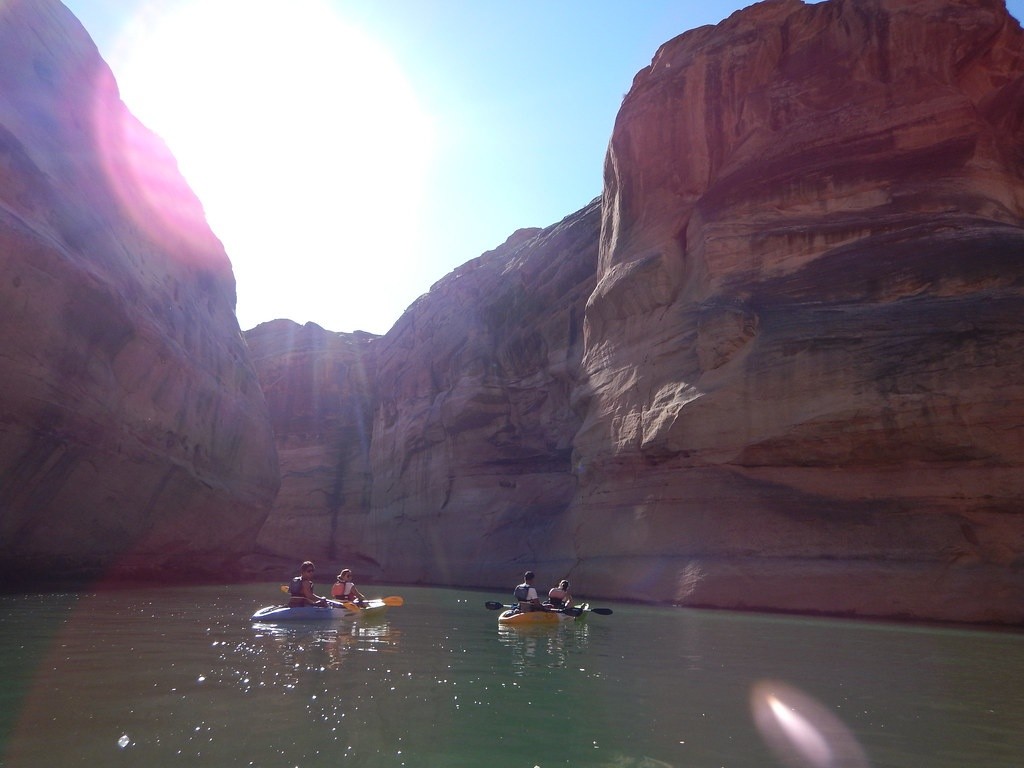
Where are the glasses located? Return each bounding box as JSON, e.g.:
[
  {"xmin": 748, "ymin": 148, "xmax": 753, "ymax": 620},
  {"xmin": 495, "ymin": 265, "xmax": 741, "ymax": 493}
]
[
  {"xmin": 345, "ymin": 575, "xmax": 352, "ymax": 578},
  {"xmin": 305, "ymin": 569, "xmax": 314, "ymax": 573}
]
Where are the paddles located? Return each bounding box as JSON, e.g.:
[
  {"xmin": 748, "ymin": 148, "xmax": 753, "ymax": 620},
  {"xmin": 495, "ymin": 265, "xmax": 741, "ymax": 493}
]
[
  {"xmin": 353, "ymin": 596, "xmax": 405, "ymax": 607},
  {"xmin": 485, "ymin": 601, "xmax": 583, "ymax": 617},
  {"xmin": 583, "ymin": 608, "xmax": 613, "ymax": 616},
  {"xmin": 281, "ymin": 585, "xmax": 359, "ymax": 611}
]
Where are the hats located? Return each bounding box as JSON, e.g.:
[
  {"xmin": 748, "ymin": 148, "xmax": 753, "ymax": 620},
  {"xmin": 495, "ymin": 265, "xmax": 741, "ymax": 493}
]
[
  {"xmin": 523, "ymin": 571, "xmax": 534, "ymax": 577},
  {"xmin": 561, "ymin": 580, "xmax": 568, "ymax": 586}
]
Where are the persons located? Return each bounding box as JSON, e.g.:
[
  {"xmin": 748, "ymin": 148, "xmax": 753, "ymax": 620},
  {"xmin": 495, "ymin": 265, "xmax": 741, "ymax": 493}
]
[
  {"xmin": 549, "ymin": 580, "xmax": 574, "ymax": 609},
  {"xmin": 288, "ymin": 561, "xmax": 327, "ymax": 607},
  {"xmin": 331, "ymin": 569, "xmax": 364, "ymax": 608},
  {"xmin": 514, "ymin": 571, "xmax": 552, "ymax": 613}
]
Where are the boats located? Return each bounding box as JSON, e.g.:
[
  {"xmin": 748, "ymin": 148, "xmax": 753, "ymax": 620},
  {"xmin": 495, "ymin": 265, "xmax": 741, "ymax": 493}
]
[
  {"xmin": 251, "ymin": 595, "xmax": 348, "ymax": 623},
  {"xmin": 496, "ymin": 608, "xmax": 577, "ymax": 626},
  {"xmin": 338, "ymin": 600, "xmax": 369, "ymax": 614}
]
